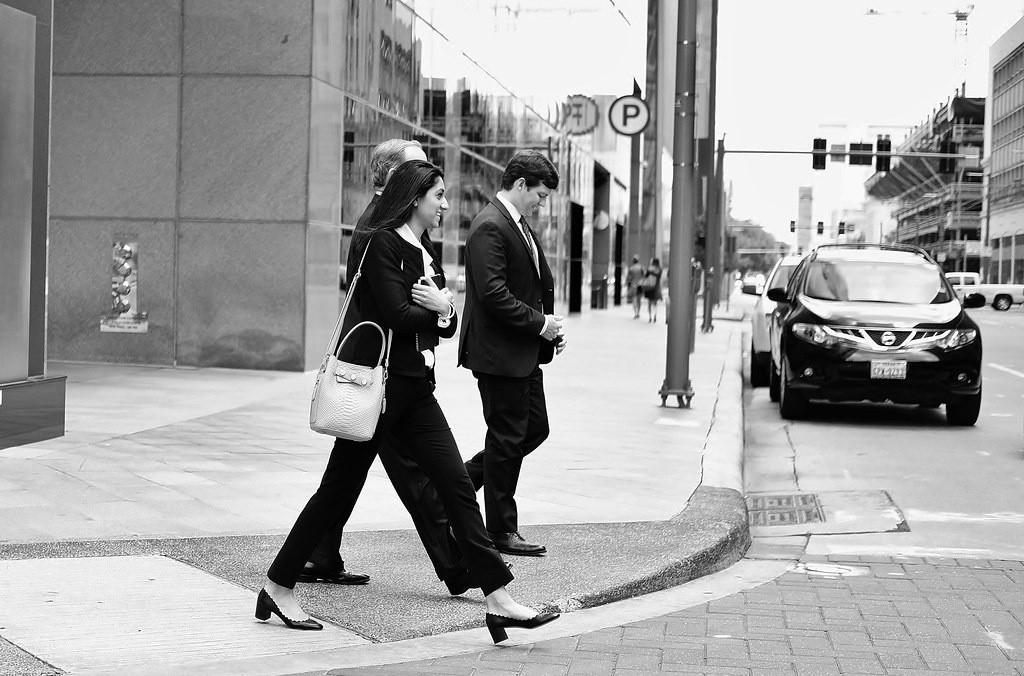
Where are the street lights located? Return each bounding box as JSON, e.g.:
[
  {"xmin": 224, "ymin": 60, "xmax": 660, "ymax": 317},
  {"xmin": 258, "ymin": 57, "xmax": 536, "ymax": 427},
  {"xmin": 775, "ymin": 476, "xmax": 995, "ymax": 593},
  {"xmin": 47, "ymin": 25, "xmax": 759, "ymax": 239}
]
[
  {"xmin": 924, "ymin": 193, "xmax": 944, "ymax": 272},
  {"xmin": 967, "ymin": 173, "xmax": 991, "ymax": 284}
]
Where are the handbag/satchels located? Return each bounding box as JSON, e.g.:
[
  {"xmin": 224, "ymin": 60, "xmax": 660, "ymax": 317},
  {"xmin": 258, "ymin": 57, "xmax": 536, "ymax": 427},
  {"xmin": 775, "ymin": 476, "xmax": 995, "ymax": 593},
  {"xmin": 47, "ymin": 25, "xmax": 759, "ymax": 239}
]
[{"xmin": 310, "ymin": 321, "xmax": 390, "ymax": 442}]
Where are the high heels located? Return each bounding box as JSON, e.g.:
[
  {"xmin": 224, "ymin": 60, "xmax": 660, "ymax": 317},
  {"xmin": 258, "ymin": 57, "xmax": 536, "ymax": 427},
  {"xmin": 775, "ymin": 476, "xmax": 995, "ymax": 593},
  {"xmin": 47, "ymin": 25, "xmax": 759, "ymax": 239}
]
[
  {"xmin": 486, "ymin": 611, "xmax": 560, "ymax": 645},
  {"xmin": 255, "ymin": 587, "xmax": 323, "ymax": 630}
]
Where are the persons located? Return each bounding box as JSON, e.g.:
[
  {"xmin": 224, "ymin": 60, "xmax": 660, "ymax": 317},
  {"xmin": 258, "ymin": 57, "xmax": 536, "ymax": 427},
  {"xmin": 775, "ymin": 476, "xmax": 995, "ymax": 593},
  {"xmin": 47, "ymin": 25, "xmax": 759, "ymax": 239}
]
[
  {"xmin": 298, "ymin": 139, "xmax": 512, "ymax": 595},
  {"xmin": 626, "ymin": 255, "xmax": 645, "ymax": 318},
  {"xmin": 255, "ymin": 159, "xmax": 559, "ymax": 645},
  {"xmin": 645, "ymin": 259, "xmax": 662, "ymax": 322},
  {"xmin": 457, "ymin": 150, "xmax": 565, "ymax": 555}
]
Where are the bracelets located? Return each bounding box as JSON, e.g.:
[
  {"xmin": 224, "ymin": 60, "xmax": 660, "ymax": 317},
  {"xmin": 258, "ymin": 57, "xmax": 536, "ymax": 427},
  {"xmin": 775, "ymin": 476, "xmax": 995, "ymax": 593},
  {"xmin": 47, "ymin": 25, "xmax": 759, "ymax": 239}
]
[{"xmin": 437, "ymin": 303, "xmax": 455, "ymax": 319}]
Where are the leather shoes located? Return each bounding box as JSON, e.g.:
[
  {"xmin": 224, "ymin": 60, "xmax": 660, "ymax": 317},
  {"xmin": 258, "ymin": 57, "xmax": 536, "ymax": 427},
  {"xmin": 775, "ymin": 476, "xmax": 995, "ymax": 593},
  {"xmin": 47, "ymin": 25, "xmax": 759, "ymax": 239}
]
[
  {"xmin": 444, "ymin": 561, "xmax": 513, "ymax": 597},
  {"xmin": 298, "ymin": 561, "xmax": 370, "ymax": 584},
  {"xmin": 487, "ymin": 530, "xmax": 548, "ymax": 554}
]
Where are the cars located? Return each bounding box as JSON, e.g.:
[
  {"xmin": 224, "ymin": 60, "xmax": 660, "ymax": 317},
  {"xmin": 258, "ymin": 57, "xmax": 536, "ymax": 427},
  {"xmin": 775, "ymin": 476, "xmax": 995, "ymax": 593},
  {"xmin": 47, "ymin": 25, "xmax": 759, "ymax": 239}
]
[
  {"xmin": 767, "ymin": 243, "xmax": 986, "ymax": 428},
  {"xmin": 741, "ymin": 271, "xmax": 765, "ymax": 289},
  {"xmin": 742, "ymin": 256, "xmax": 808, "ymax": 389}
]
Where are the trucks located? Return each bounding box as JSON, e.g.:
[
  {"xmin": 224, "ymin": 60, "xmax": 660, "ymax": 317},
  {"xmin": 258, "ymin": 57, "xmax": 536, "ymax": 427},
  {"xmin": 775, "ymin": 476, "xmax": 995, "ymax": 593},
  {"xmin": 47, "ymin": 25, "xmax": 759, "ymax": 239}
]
[{"xmin": 945, "ymin": 272, "xmax": 1024, "ymax": 312}]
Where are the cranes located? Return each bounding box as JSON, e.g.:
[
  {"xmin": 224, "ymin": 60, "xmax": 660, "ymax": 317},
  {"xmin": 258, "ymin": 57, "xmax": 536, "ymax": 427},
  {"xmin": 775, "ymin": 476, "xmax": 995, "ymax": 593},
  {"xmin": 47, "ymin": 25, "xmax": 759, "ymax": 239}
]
[{"xmin": 865, "ymin": 3, "xmax": 974, "ymax": 98}]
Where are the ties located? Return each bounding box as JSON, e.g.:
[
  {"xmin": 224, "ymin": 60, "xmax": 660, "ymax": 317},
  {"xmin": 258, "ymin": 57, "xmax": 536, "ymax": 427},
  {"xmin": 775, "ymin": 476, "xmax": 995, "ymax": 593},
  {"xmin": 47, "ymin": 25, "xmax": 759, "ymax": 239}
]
[{"xmin": 520, "ymin": 216, "xmax": 541, "ymax": 279}]
